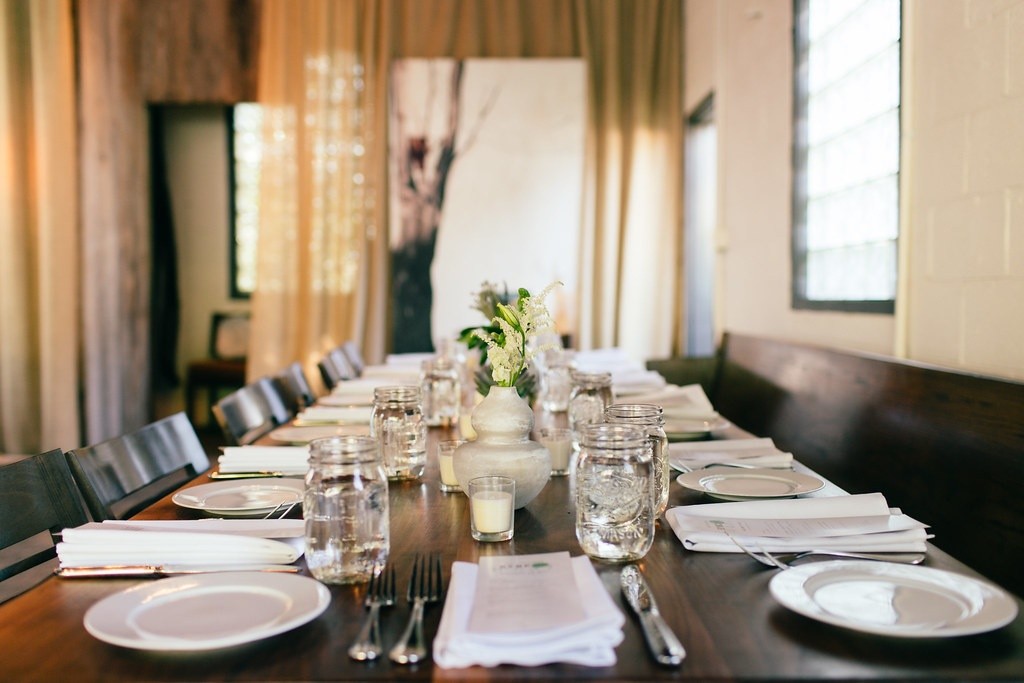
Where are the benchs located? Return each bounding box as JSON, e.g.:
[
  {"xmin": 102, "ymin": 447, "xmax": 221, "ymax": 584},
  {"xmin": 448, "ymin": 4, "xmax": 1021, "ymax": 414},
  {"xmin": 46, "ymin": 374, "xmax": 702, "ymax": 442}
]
[{"xmin": 647, "ymin": 329, "xmax": 1024, "ymax": 601}]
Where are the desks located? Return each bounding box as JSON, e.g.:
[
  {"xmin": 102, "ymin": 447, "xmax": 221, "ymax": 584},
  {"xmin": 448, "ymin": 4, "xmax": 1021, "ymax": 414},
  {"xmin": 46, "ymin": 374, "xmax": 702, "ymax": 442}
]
[{"xmin": 0, "ymin": 359, "xmax": 1024, "ymax": 682}]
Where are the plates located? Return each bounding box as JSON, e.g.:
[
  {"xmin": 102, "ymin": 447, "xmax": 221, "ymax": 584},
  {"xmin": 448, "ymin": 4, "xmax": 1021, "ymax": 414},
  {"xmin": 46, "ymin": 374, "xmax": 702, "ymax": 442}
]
[
  {"xmin": 664, "ymin": 415, "xmax": 731, "ymax": 439},
  {"xmin": 768, "ymin": 560, "xmax": 1018, "ymax": 637},
  {"xmin": 83, "ymin": 571, "xmax": 331, "ymax": 652},
  {"xmin": 269, "ymin": 426, "xmax": 370, "ymax": 445},
  {"xmin": 679, "ymin": 469, "xmax": 825, "ymax": 501},
  {"xmin": 172, "ymin": 478, "xmax": 304, "ymax": 515}
]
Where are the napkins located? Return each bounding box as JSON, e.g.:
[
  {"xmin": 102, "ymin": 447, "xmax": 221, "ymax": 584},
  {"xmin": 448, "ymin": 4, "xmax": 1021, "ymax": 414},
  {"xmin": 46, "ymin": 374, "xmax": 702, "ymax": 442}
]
[
  {"xmin": 56, "ymin": 516, "xmax": 308, "ymax": 566},
  {"xmin": 294, "ymin": 349, "xmax": 937, "ymax": 667},
  {"xmin": 218, "ymin": 445, "xmax": 308, "ymax": 473}
]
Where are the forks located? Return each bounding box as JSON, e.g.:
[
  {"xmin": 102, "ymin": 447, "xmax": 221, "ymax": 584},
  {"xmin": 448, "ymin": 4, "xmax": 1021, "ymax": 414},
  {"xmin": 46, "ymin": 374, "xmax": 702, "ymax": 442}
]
[
  {"xmin": 389, "ymin": 551, "xmax": 443, "ymax": 663},
  {"xmin": 730, "ymin": 536, "xmax": 925, "ymax": 566},
  {"xmin": 348, "ymin": 559, "xmax": 395, "ymax": 659}
]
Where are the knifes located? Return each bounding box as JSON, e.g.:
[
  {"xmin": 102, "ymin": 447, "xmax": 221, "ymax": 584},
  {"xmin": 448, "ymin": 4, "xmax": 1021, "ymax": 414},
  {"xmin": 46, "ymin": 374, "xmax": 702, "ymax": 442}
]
[
  {"xmin": 619, "ymin": 565, "xmax": 686, "ymax": 664},
  {"xmin": 210, "ymin": 470, "xmax": 308, "ymax": 477}
]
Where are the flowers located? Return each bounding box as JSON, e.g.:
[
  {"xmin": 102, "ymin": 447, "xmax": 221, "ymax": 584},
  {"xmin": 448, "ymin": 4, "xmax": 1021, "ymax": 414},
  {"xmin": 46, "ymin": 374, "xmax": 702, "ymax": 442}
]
[{"xmin": 456, "ymin": 274, "xmax": 566, "ymax": 398}]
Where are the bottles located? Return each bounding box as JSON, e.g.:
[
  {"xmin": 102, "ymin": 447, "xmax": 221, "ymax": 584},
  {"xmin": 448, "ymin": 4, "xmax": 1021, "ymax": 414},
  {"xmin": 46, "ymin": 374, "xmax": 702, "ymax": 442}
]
[
  {"xmin": 303, "ymin": 436, "xmax": 390, "ymax": 583},
  {"xmin": 420, "ymin": 359, "xmax": 461, "ymax": 426},
  {"xmin": 575, "ymin": 423, "xmax": 655, "ymax": 558},
  {"xmin": 568, "ymin": 371, "xmax": 614, "ymax": 451},
  {"xmin": 602, "ymin": 403, "xmax": 670, "ymax": 519},
  {"xmin": 370, "ymin": 386, "xmax": 426, "ymax": 480},
  {"xmin": 542, "ymin": 365, "xmax": 576, "ymax": 411}
]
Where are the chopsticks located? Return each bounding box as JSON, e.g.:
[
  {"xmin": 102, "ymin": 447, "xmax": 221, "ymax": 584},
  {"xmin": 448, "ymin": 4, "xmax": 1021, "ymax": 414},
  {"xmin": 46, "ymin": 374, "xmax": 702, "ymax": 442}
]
[{"xmin": 53, "ymin": 565, "xmax": 303, "ymax": 577}]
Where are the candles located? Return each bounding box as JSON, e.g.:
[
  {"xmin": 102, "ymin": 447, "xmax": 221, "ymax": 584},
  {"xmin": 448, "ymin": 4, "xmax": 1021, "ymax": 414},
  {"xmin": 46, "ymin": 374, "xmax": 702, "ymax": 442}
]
[{"xmin": 473, "ymin": 491, "xmax": 513, "ymax": 534}]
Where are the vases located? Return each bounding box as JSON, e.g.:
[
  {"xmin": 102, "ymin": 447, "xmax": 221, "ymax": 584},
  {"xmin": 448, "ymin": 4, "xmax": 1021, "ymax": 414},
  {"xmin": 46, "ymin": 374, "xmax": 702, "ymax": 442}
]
[{"xmin": 453, "ymin": 386, "xmax": 552, "ymax": 513}]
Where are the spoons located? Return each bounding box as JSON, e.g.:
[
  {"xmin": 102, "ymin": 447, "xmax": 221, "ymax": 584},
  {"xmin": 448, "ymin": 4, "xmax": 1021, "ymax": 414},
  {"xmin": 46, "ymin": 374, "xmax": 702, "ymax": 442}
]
[{"xmin": 670, "ymin": 462, "xmax": 794, "ymax": 473}]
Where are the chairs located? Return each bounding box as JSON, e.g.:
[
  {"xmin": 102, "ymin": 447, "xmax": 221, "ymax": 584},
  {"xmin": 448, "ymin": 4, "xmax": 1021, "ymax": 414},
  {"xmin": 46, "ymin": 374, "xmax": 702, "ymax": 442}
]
[
  {"xmin": 0, "ymin": 347, "xmax": 377, "ymax": 603},
  {"xmin": 183, "ymin": 309, "xmax": 249, "ymax": 451}
]
